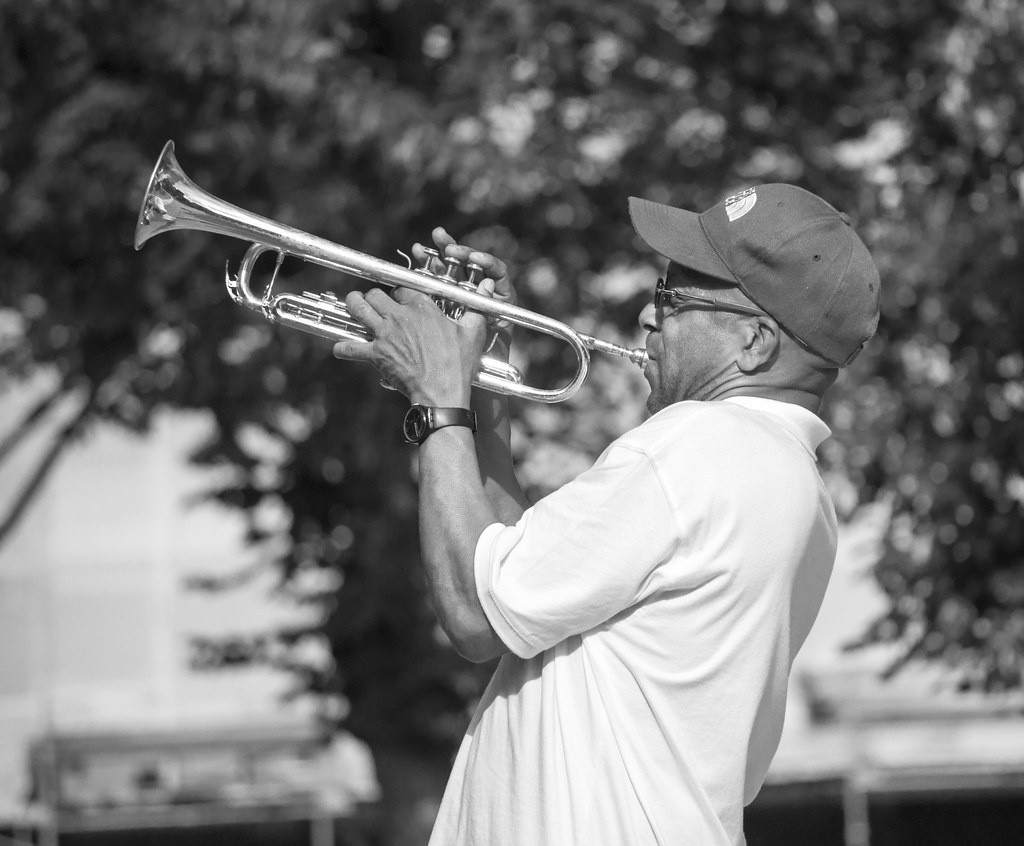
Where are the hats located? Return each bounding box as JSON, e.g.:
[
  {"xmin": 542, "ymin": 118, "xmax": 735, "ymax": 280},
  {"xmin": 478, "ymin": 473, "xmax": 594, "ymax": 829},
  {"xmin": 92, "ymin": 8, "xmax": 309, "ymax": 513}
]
[{"xmin": 627, "ymin": 183, "xmax": 882, "ymax": 368}]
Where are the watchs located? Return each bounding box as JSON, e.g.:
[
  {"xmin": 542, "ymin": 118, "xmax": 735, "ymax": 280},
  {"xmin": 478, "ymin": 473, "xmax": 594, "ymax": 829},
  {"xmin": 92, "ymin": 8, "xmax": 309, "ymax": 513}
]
[{"xmin": 402, "ymin": 403, "xmax": 479, "ymax": 444}]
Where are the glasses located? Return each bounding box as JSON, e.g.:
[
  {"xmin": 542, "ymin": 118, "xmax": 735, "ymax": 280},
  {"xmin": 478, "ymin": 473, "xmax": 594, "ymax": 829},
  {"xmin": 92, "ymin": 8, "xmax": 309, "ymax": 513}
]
[{"xmin": 654, "ymin": 277, "xmax": 767, "ymax": 319}]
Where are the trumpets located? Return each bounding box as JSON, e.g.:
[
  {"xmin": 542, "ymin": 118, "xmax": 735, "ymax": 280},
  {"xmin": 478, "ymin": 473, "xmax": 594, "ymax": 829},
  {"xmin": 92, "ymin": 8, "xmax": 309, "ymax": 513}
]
[{"xmin": 134, "ymin": 138, "xmax": 648, "ymax": 404}]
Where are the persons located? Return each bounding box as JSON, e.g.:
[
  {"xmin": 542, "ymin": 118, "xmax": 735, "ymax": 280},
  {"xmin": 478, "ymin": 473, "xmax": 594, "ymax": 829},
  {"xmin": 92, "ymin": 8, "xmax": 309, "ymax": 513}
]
[{"xmin": 333, "ymin": 185, "xmax": 883, "ymax": 846}]
